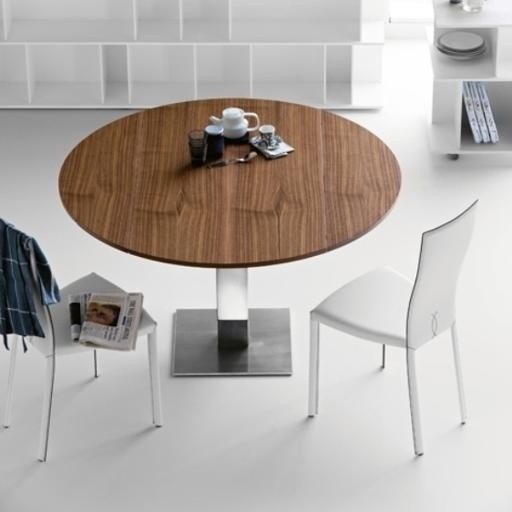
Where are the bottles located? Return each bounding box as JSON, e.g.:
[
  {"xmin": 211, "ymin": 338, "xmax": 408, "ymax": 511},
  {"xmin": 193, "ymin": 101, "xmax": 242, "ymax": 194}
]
[{"xmin": 203, "ymin": 125, "xmax": 224, "ymax": 162}]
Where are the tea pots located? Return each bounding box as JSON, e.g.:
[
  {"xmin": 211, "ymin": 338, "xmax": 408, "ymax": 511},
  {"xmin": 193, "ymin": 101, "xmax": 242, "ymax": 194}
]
[{"xmin": 210, "ymin": 108, "xmax": 260, "ymax": 139}]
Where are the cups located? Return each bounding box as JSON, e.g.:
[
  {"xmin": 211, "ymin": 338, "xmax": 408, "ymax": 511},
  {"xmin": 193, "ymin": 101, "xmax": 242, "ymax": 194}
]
[
  {"xmin": 463, "ymin": 0, "xmax": 483, "ymax": 15},
  {"xmin": 188, "ymin": 129, "xmax": 206, "ymax": 165},
  {"xmin": 259, "ymin": 124, "xmax": 275, "ymax": 150}
]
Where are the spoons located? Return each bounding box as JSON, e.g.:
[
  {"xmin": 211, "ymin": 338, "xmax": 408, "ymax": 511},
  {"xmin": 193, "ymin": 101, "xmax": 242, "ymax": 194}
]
[{"xmin": 210, "ymin": 151, "xmax": 261, "ymax": 168}]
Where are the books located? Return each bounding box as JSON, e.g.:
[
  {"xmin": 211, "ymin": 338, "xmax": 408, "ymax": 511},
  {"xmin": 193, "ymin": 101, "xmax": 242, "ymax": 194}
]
[{"xmin": 463, "ymin": 81, "xmax": 500, "ymax": 143}]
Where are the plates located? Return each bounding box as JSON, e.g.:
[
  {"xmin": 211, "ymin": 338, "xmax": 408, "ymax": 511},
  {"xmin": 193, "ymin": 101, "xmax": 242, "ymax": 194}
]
[{"xmin": 434, "ymin": 32, "xmax": 490, "ymax": 57}]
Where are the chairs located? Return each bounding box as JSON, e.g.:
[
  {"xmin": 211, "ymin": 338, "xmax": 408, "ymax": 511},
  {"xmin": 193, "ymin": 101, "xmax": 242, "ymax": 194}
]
[
  {"xmin": 0, "ymin": 218, "xmax": 164, "ymax": 463},
  {"xmin": 308, "ymin": 198, "xmax": 480, "ymax": 456}
]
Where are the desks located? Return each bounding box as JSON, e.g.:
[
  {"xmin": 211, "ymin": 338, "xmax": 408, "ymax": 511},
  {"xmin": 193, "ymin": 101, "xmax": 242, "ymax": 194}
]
[{"xmin": 57, "ymin": 97, "xmax": 403, "ymax": 378}]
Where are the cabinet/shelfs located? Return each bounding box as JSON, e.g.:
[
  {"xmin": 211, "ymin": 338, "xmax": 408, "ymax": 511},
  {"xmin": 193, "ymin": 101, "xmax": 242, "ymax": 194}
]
[
  {"xmin": 1, "ymin": 0, "xmax": 386, "ymax": 114},
  {"xmin": 424, "ymin": 0, "xmax": 512, "ymax": 160}
]
